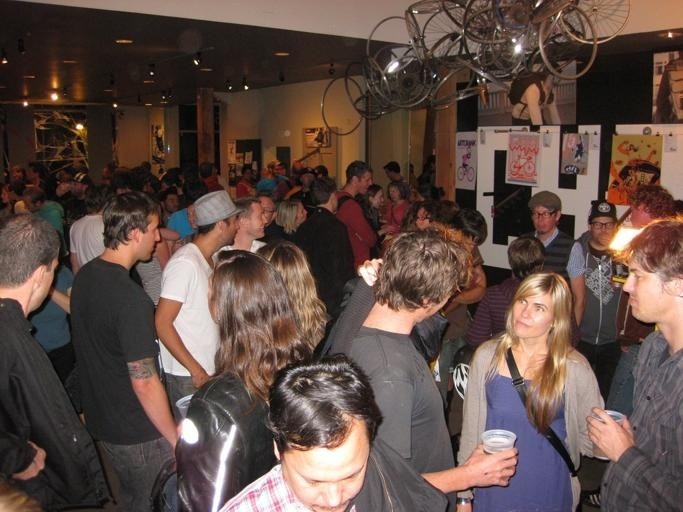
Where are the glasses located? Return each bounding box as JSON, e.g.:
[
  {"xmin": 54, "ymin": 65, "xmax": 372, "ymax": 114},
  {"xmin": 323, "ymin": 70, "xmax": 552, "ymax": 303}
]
[
  {"xmin": 532, "ymin": 209, "xmax": 557, "ymax": 218},
  {"xmin": 590, "ymin": 222, "xmax": 616, "ymax": 230}
]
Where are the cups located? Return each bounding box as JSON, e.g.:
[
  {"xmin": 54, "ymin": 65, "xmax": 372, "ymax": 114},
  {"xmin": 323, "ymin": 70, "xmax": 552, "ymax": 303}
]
[
  {"xmin": 176, "ymin": 394, "xmax": 194, "ymax": 418},
  {"xmin": 481, "ymin": 430, "xmax": 516, "ymax": 455},
  {"xmin": 591, "ymin": 411, "xmax": 624, "ymax": 461}
]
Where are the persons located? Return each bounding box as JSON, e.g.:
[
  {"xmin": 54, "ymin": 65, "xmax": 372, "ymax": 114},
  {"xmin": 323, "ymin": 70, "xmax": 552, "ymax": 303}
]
[
  {"xmin": 510, "ymin": 62, "xmax": 561, "ymax": 128},
  {"xmin": 1, "ymin": 159, "xmax": 682, "ymax": 512}
]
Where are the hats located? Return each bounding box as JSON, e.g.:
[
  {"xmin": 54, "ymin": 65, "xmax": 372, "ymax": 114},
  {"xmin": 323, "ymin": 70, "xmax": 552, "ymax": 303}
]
[
  {"xmin": 528, "ymin": 191, "xmax": 561, "ymax": 209},
  {"xmin": 589, "ymin": 202, "xmax": 617, "ymax": 221},
  {"xmin": 193, "ymin": 190, "xmax": 242, "ymax": 227}
]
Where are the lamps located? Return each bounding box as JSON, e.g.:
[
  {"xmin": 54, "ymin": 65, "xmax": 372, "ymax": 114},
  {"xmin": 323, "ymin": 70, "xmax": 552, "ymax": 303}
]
[{"xmin": 147, "ymin": 53, "xmax": 250, "ymax": 105}]
